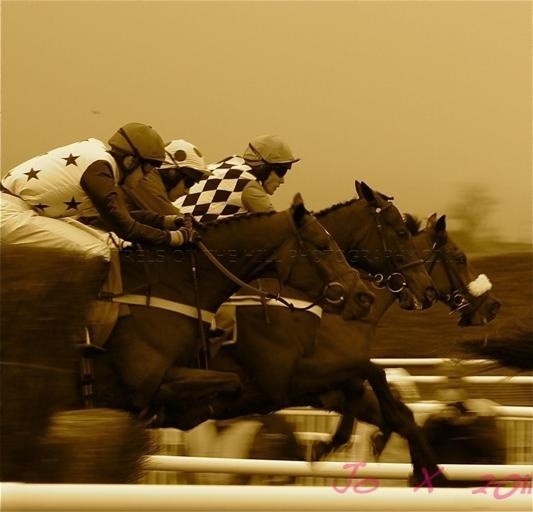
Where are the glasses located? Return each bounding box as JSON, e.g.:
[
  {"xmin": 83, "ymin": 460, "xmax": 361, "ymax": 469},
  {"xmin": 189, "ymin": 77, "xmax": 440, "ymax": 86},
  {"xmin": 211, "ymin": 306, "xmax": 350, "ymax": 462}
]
[
  {"xmin": 182, "ymin": 173, "xmax": 195, "ymax": 190},
  {"xmin": 272, "ymin": 165, "xmax": 288, "ymax": 179},
  {"xmin": 140, "ymin": 160, "xmax": 155, "ymax": 176}
]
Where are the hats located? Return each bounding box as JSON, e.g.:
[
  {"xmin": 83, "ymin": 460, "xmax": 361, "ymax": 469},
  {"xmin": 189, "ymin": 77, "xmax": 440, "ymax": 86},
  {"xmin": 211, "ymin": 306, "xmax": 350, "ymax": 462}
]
[
  {"xmin": 243, "ymin": 135, "xmax": 300, "ymax": 168},
  {"xmin": 108, "ymin": 120, "xmax": 174, "ymax": 166},
  {"xmin": 156, "ymin": 139, "xmax": 215, "ymax": 178}
]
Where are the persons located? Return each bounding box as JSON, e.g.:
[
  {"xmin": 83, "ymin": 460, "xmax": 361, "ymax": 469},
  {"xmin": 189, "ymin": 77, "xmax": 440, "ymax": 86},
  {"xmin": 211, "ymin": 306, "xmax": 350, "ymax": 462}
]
[
  {"xmin": 77, "ymin": 139, "xmax": 215, "ymax": 233},
  {"xmin": 170, "ymin": 136, "xmax": 300, "ymax": 360},
  {"xmin": 0, "ymin": 123, "xmax": 203, "ymax": 263}
]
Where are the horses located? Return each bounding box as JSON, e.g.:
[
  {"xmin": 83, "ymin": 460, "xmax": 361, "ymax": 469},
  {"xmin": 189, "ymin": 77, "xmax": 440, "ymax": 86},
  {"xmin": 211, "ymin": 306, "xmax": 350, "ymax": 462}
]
[{"xmin": 0, "ymin": 180, "xmax": 502, "ymax": 488}]
